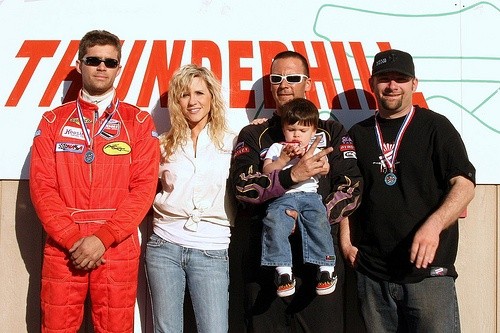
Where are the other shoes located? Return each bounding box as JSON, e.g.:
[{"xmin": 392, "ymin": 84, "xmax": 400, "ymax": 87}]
[
  {"xmin": 277, "ymin": 273, "xmax": 296, "ymax": 297},
  {"xmin": 316, "ymin": 270, "xmax": 338, "ymax": 295}
]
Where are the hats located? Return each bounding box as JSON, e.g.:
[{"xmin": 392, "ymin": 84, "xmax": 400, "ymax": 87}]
[{"xmin": 373, "ymin": 50, "xmax": 416, "ymax": 78}]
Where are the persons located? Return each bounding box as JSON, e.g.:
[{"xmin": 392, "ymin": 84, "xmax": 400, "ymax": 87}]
[
  {"xmin": 144, "ymin": 64, "xmax": 268, "ymax": 333},
  {"xmin": 340, "ymin": 49, "xmax": 476, "ymax": 333},
  {"xmin": 226, "ymin": 51, "xmax": 364, "ymax": 333},
  {"xmin": 28, "ymin": 29, "xmax": 160, "ymax": 333}
]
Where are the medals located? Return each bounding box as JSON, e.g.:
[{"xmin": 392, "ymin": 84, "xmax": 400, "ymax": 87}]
[
  {"xmin": 83, "ymin": 150, "xmax": 95, "ymax": 163},
  {"xmin": 385, "ymin": 173, "xmax": 396, "ymax": 185}
]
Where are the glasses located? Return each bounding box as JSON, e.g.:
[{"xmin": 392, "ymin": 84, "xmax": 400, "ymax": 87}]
[
  {"xmin": 269, "ymin": 74, "xmax": 308, "ymax": 84},
  {"xmin": 81, "ymin": 56, "xmax": 120, "ymax": 68}
]
